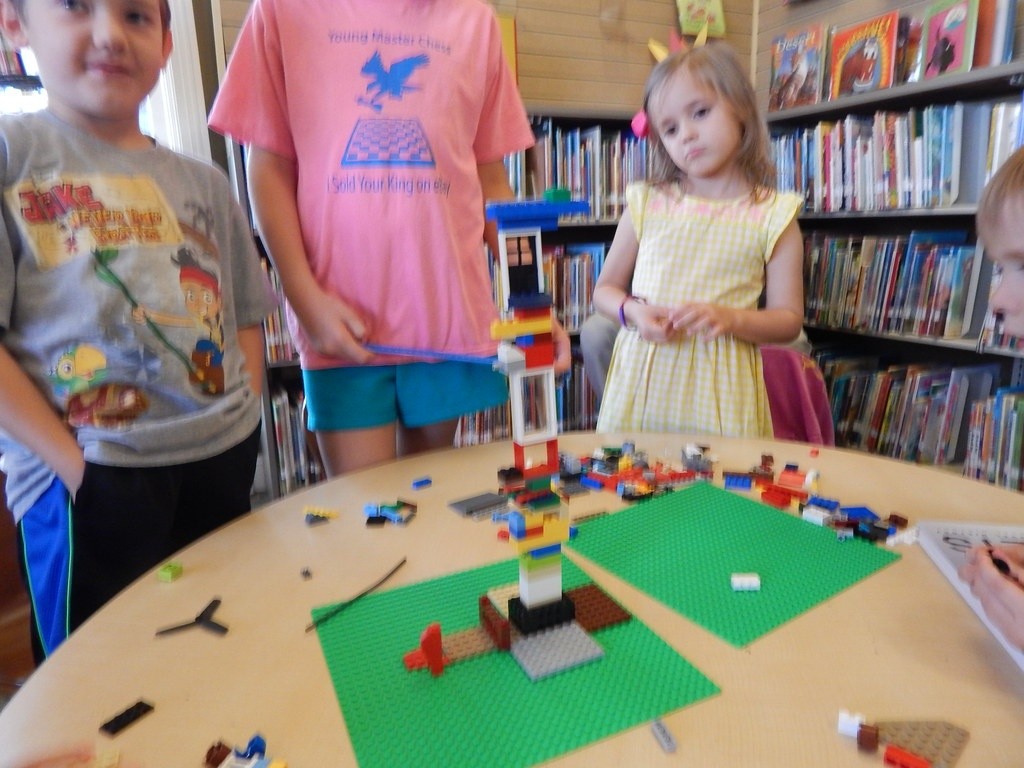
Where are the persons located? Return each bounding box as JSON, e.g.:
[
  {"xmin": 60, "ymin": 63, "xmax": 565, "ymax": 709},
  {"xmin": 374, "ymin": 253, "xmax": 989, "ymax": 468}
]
[
  {"xmin": 0, "ymin": 0, "xmax": 271, "ymax": 669},
  {"xmin": 961, "ymin": 146, "xmax": 1024, "ymax": 656},
  {"xmin": 206, "ymin": 0, "xmax": 570, "ymax": 483},
  {"xmin": 594, "ymin": 40, "xmax": 805, "ymax": 439}
]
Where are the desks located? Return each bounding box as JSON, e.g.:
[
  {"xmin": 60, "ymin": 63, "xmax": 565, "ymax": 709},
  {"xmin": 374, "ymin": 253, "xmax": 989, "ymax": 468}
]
[{"xmin": 0, "ymin": 431, "xmax": 1024, "ymax": 768}]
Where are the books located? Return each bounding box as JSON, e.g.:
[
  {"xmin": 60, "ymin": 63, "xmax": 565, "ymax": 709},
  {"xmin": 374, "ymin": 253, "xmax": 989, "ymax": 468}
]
[
  {"xmin": 216, "ymin": 2, "xmax": 1024, "ymax": 504},
  {"xmin": 916, "ymin": 515, "xmax": 1024, "ymax": 677}
]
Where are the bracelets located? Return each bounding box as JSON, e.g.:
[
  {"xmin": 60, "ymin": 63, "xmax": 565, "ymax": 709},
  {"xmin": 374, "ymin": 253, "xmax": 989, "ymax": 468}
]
[{"xmin": 618, "ymin": 296, "xmax": 647, "ymax": 328}]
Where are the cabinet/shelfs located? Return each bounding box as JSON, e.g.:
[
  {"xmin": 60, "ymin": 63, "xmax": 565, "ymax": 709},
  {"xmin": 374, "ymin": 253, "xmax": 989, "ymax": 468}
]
[{"xmin": 225, "ymin": 61, "xmax": 1024, "ymax": 512}]
